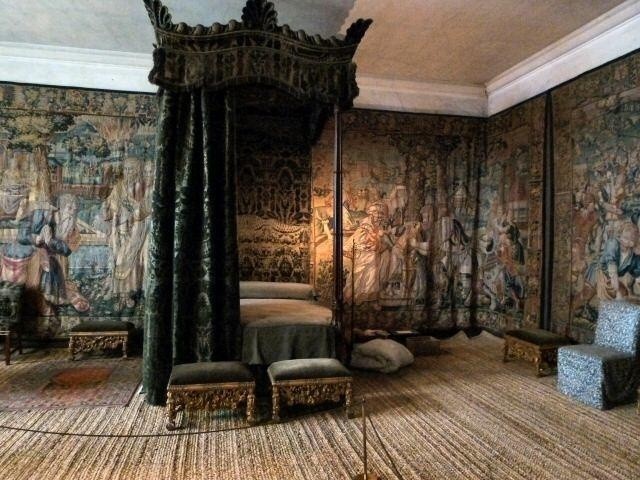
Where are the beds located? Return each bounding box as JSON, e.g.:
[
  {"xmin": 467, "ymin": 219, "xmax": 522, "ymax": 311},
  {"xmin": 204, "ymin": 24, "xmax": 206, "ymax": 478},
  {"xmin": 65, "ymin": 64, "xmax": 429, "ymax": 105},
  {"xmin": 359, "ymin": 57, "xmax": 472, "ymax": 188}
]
[{"xmin": 138, "ymin": 1, "xmax": 371, "ymax": 404}]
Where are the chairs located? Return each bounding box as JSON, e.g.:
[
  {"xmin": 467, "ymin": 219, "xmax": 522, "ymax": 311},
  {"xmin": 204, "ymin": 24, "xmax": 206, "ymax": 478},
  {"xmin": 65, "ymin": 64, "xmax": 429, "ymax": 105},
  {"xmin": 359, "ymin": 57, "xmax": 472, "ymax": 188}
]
[{"xmin": 552, "ymin": 300, "xmax": 640, "ymax": 411}]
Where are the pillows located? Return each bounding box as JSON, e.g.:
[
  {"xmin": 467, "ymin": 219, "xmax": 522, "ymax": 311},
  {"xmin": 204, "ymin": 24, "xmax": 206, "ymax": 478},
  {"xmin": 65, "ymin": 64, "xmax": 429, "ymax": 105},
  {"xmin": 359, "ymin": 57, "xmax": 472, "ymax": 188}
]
[{"xmin": 239, "ymin": 281, "xmax": 317, "ymax": 301}]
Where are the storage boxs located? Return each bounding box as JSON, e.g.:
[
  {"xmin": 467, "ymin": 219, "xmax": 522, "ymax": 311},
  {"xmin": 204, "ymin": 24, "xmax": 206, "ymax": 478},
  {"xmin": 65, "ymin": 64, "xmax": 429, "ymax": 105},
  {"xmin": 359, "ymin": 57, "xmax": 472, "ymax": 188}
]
[{"xmin": 407, "ymin": 336, "xmax": 441, "ymax": 356}]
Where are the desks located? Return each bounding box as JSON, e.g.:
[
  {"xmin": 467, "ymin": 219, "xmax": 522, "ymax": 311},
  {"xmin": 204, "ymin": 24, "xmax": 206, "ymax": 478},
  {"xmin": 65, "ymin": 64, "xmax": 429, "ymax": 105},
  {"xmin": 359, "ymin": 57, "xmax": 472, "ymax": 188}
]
[{"xmin": 0, "ymin": 279, "xmax": 27, "ymax": 366}]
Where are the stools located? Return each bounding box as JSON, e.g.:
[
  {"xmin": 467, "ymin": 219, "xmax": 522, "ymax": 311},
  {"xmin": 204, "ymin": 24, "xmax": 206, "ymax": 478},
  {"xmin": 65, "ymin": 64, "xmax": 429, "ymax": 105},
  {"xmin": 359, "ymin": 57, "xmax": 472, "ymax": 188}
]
[
  {"xmin": 502, "ymin": 326, "xmax": 573, "ymax": 377},
  {"xmin": 68, "ymin": 321, "xmax": 133, "ymax": 360},
  {"xmin": 163, "ymin": 360, "xmax": 257, "ymax": 430},
  {"xmin": 265, "ymin": 357, "xmax": 351, "ymax": 420}
]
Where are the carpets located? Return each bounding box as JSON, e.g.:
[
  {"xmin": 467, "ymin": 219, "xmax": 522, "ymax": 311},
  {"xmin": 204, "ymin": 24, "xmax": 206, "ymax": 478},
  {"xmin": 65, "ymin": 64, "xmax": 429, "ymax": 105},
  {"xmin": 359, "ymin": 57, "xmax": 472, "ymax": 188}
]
[{"xmin": 1, "ymin": 359, "xmax": 139, "ymax": 407}]
